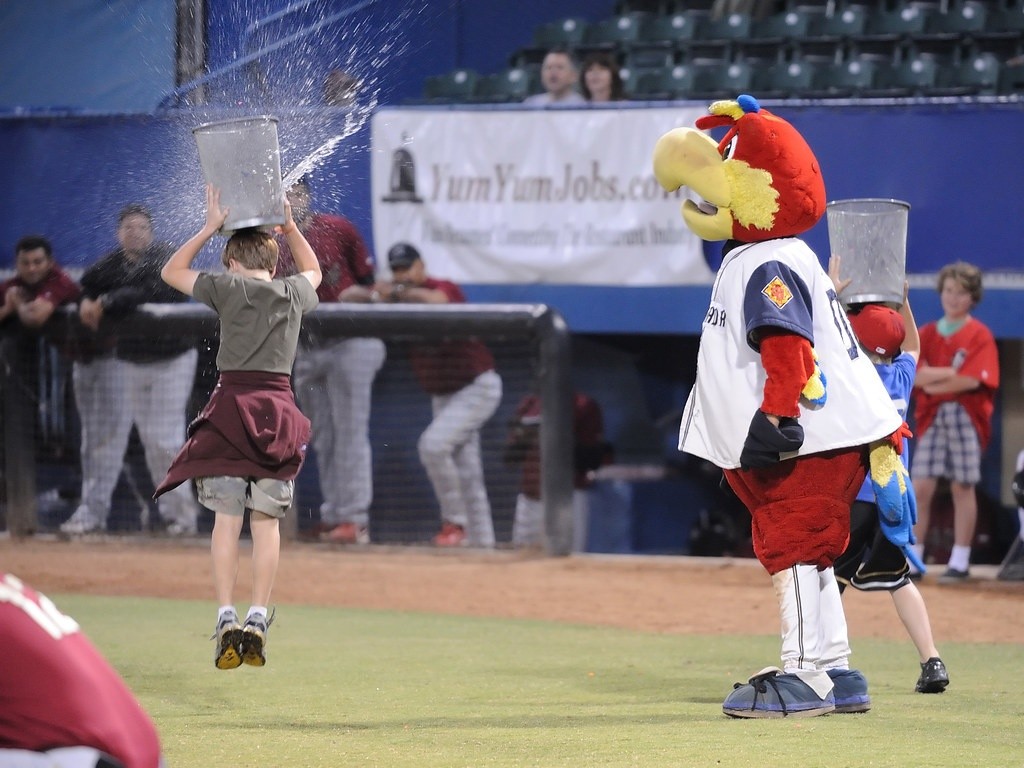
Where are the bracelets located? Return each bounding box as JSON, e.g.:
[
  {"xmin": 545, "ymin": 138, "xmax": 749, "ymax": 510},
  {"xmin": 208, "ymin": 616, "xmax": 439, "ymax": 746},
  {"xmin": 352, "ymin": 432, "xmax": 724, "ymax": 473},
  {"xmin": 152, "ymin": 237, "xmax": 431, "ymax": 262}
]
[{"xmin": 391, "ymin": 283, "xmax": 410, "ymax": 302}]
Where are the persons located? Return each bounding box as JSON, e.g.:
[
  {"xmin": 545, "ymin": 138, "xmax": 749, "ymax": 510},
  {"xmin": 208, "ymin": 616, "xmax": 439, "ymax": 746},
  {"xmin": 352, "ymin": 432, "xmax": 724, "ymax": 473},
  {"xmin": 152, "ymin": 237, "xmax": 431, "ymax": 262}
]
[
  {"xmin": 60, "ymin": 206, "xmax": 199, "ymax": 535},
  {"xmin": 371, "ymin": 243, "xmax": 503, "ymax": 548},
  {"xmin": 504, "ymin": 369, "xmax": 617, "ymax": 553},
  {"xmin": 825, "ymin": 253, "xmax": 950, "ymax": 694},
  {"xmin": 152, "ymin": 182, "xmax": 323, "ymax": 670},
  {"xmin": 906, "ymin": 261, "xmax": 998, "ymax": 585},
  {"xmin": 0, "ymin": 568, "xmax": 166, "ymax": 768},
  {"xmin": 272, "ymin": 172, "xmax": 386, "ymax": 544},
  {"xmin": 520, "ymin": 47, "xmax": 632, "ymax": 103},
  {"xmin": 0, "ymin": 236, "xmax": 84, "ymax": 513}
]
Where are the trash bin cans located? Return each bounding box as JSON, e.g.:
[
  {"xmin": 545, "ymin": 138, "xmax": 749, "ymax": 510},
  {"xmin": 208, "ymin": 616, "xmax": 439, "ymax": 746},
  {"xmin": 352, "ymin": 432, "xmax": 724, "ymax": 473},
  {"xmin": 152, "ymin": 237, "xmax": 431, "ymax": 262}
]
[
  {"xmin": 827, "ymin": 198, "xmax": 909, "ymax": 306},
  {"xmin": 576, "ymin": 462, "xmax": 695, "ymax": 558},
  {"xmin": 190, "ymin": 115, "xmax": 287, "ymax": 233}
]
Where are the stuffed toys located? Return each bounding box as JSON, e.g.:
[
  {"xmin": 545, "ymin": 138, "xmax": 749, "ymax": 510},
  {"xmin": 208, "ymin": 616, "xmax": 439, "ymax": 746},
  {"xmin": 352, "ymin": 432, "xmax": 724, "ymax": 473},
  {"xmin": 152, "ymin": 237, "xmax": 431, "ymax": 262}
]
[{"xmin": 653, "ymin": 95, "xmax": 913, "ymax": 719}]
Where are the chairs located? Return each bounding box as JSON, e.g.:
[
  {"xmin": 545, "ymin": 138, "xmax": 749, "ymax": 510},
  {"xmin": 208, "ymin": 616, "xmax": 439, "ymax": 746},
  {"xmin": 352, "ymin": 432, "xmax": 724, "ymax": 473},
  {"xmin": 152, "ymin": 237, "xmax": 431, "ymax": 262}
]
[{"xmin": 420, "ymin": 0, "xmax": 1024, "ymax": 106}]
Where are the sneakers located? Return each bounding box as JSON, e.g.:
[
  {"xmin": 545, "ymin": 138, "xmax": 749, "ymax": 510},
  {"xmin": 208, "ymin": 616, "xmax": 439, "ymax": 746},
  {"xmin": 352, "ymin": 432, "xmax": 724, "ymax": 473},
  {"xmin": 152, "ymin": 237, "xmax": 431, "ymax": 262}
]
[
  {"xmin": 209, "ymin": 607, "xmax": 275, "ymax": 670},
  {"xmin": 60, "ymin": 505, "xmax": 107, "ymax": 536},
  {"xmin": 914, "ymin": 657, "xmax": 949, "ymax": 694},
  {"xmin": 939, "ymin": 568, "xmax": 969, "ymax": 584},
  {"xmin": 429, "ymin": 519, "xmax": 469, "ymax": 549},
  {"xmin": 319, "ymin": 522, "xmax": 370, "ymax": 544}
]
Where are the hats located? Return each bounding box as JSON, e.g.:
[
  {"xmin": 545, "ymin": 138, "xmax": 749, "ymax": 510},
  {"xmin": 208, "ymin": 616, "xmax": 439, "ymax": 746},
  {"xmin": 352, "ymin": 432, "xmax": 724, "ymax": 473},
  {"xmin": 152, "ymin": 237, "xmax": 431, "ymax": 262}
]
[{"xmin": 388, "ymin": 243, "xmax": 418, "ymax": 266}]
